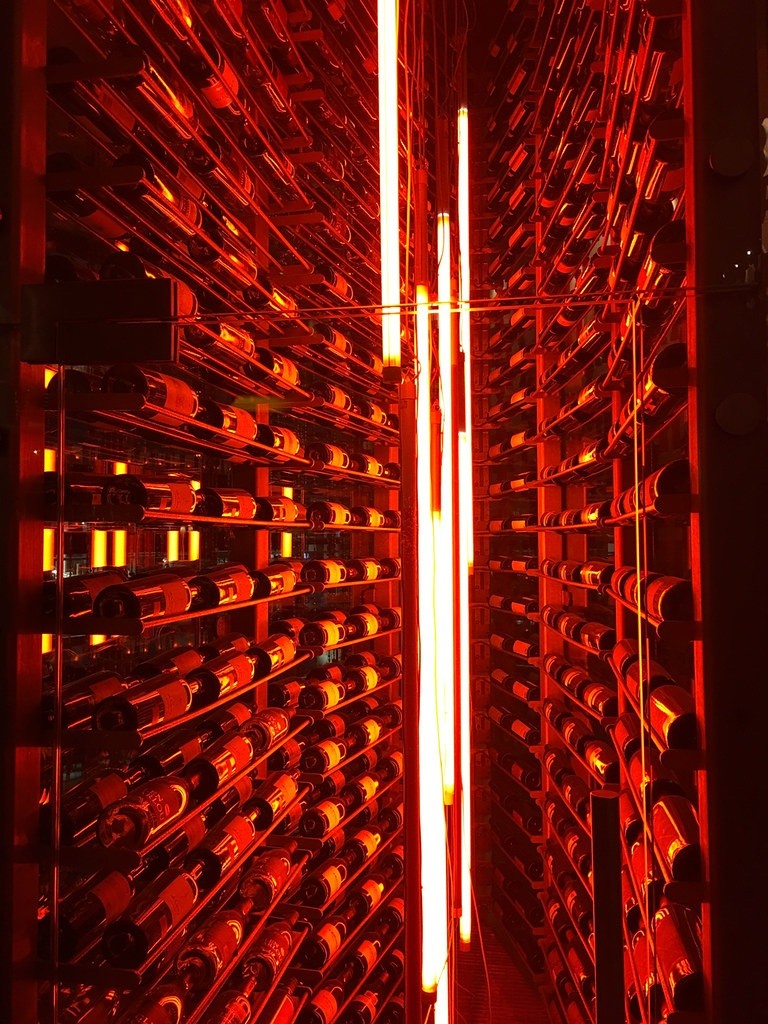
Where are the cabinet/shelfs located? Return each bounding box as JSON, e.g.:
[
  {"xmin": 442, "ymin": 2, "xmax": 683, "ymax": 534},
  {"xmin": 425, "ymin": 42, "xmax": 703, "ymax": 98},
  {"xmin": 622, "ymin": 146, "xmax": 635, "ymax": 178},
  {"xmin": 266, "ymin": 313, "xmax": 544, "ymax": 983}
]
[{"xmin": 0, "ymin": 0, "xmax": 768, "ymax": 1024}]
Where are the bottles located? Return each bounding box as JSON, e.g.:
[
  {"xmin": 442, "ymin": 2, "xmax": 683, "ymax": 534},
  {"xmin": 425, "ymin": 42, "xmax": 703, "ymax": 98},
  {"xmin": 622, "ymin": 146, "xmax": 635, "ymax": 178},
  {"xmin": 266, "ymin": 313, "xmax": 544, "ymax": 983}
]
[
  {"xmin": 41, "ymin": 0, "xmax": 439, "ymax": 1024},
  {"xmin": 442, "ymin": 0, "xmax": 713, "ymax": 1023}
]
